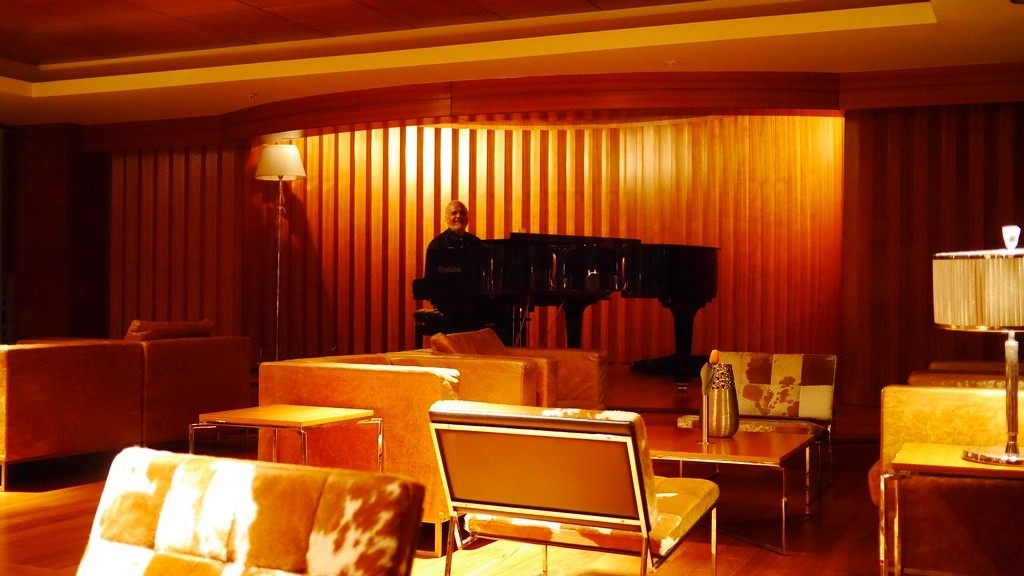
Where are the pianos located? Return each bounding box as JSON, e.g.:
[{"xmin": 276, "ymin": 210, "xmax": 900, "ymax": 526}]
[{"xmin": 412, "ymin": 229, "xmax": 722, "ymax": 358}]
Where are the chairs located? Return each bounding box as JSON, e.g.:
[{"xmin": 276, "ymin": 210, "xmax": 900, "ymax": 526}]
[
  {"xmin": 414, "ymin": 327, "xmax": 607, "ymax": 412},
  {"xmin": 0, "ymin": 339, "xmax": 143, "ymax": 491},
  {"xmin": 123, "ymin": 320, "xmax": 254, "ymax": 450},
  {"xmin": 258, "ymin": 352, "xmax": 538, "ymax": 557}
]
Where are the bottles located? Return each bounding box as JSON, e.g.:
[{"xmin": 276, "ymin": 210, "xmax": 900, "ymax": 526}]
[{"xmin": 562, "ymin": 264, "xmax": 570, "ymax": 288}]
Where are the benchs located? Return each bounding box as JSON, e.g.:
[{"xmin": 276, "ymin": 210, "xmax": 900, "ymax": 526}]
[
  {"xmin": 869, "ymin": 361, "xmax": 1024, "ymax": 576},
  {"xmin": 74, "ymin": 443, "xmax": 426, "ymax": 576},
  {"xmin": 677, "ymin": 352, "xmax": 839, "ymax": 487},
  {"xmin": 428, "ymin": 400, "xmax": 719, "ymax": 576}
]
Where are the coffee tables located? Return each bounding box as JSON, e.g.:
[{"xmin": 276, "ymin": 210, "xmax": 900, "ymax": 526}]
[{"xmin": 188, "ymin": 404, "xmax": 383, "ymax": 473}]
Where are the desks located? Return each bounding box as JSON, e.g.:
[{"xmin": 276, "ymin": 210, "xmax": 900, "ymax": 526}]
[
  {"xmin": 649, "ymin": 426, "xmax": 814, "ymax": 556},
  {"xmin": 878, "ymin": 444, "xmax": 1024, "ymax": 576}
]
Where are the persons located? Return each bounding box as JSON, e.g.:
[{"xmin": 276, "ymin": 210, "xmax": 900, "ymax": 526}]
[{"xmin": 423, "ymin": 201, "xmax": 508, "ymax": 322}]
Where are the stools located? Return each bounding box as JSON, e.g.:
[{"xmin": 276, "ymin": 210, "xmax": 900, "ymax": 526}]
[{"xmin": 413, "ymin": 310, "xmax": 444, "ymax": 351}]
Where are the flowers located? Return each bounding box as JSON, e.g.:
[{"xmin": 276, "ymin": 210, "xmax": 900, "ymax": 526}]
[{"xmin": 699, "ymin": 348, "xmax": 720, "ymax": 395}]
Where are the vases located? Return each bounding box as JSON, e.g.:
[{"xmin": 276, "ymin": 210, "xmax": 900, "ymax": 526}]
[
  {"xmin": 697, "ymin": 394, "xmax": 716, "ymax": 446},
  {"xmin": 706, "ymin": 364, "xmax": 740, "ymax": 438}
]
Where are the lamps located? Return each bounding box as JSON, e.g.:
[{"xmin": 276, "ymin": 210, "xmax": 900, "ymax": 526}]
[
  {"xmin": 933, "ymin": 224, "xmax": 1024, "ymax": 465},
  {"xmin": 251, "ymin": 144, "xmax": 307, "ymax": 363}
]
